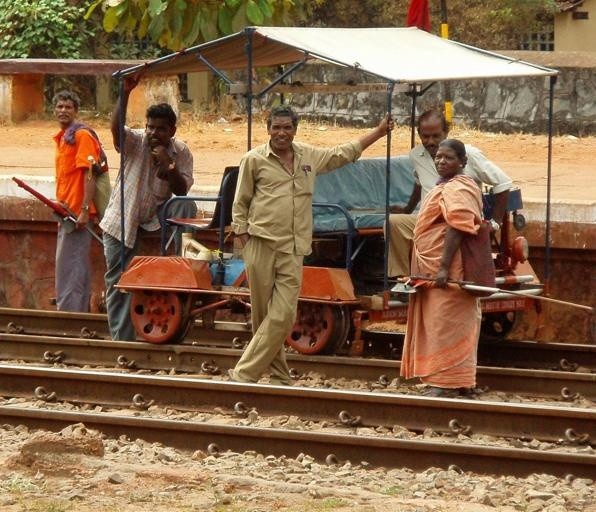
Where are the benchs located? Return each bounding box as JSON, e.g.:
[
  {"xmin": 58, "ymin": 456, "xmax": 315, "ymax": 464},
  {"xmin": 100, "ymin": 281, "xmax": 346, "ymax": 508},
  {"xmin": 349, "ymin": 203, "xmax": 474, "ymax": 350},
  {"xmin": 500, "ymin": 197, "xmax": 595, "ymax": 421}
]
[
  {"xmin": 159, "ymin": 166, "xmax": 240, "ymax": 285},
  {"xmin": 312, "ymin": 156, "xmax": 423, "ymax": 270}
]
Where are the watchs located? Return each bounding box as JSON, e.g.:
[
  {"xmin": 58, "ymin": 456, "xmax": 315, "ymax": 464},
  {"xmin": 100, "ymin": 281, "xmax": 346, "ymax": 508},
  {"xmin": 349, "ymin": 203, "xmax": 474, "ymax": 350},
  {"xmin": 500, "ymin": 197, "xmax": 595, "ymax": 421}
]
[{"xmin": 489, "ymin": 217, "xmax": 500, "ymax": 231}]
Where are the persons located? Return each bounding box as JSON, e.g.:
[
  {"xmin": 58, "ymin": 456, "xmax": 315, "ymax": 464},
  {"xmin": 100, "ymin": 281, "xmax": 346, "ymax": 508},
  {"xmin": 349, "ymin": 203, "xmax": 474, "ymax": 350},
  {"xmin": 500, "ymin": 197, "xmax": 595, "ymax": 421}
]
[
  {"xmin": 47, "ymin": 88, "xmax": 102, "ymax": 314},
  {"xmin": 224, "ymin": 103, "xmax": 396, "ymax": 386},
  {"xmin": 94, "ymin": 71, "xmax": 200, "ymax": 345},
  {"xmin": 377, "ymin": 108, "xmax": 515, "ymax": 311},
  {"xmin": 392, "ymin": 137, "xmax": 486, "ymax": 400}
]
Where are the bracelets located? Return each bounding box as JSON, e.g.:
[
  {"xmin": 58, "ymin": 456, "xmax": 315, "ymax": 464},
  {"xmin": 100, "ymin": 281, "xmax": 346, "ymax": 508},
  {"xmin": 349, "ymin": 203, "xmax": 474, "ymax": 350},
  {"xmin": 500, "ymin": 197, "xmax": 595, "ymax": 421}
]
[
  {"xmin": 79, "ymin": 204, "xmax": 89, "ymax": 210},
  {"xmin": 164, "ymin": 161, "xmax": 175, "ymax": 173}
]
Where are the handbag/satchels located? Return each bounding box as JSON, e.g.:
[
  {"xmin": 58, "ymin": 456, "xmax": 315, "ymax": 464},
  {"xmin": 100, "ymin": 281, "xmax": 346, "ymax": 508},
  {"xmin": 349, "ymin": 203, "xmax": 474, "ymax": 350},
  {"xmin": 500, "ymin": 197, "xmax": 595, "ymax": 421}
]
[
  {"xmin": 92, "ymin": 151, "xmax": 112, "ymax": 219},
  {"xmin": 461, "ymin": 221, "xmax": 496, "ymax": 297}
]
